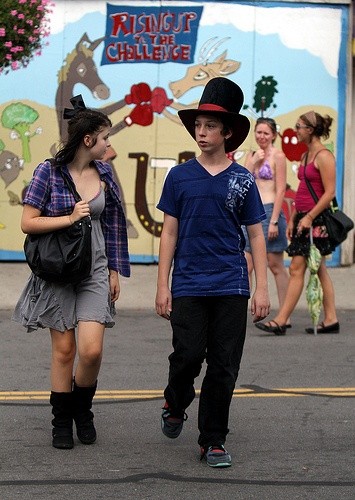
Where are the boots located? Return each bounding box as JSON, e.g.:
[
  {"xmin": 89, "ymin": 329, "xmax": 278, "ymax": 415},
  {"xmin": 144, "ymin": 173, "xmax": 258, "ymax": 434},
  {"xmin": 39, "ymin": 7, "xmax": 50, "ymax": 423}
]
[
  {"xmin": 74, "ymin": 376, "xmax": 98, "ymax": 444},
  {"xmin": 50, "ymin": 391, "xmax": 74, "ymax": 449}
]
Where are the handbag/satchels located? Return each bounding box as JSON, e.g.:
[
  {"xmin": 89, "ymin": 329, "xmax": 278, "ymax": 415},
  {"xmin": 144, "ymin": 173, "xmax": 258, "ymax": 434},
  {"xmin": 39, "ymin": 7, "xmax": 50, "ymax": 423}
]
[
  {"xmin": 23, "ymin": 217, "xmax": 93, "ymax": 285},
  {"xmin": 325, "ymin": 210, "xmax": 354, "ymax": 245}
]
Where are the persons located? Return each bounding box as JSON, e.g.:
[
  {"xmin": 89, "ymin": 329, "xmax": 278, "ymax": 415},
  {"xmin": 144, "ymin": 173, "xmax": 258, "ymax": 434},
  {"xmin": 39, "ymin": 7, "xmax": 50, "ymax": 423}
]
[
  {"xmin": 155, "ymin": 77, "xmax": 271, "ymax": 467},
  {"xmin": 11, "ymin": 94, "xmax": 132, "ymax": 449},
  {"xmin": 241, "ymin": 111, "xmax": 342, "ymax": 333}
]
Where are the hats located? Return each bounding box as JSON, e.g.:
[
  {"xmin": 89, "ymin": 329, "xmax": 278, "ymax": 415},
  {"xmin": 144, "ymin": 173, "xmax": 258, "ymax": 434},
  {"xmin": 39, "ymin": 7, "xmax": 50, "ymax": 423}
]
[{"xmin": 178, "ymin": 78, "xmax": 251, "ymax": 153}]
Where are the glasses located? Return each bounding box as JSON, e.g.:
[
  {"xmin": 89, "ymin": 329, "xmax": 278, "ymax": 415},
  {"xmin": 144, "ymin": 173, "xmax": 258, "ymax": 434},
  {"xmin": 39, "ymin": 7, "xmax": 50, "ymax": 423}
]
[
  {"xmin": 258, "ymin": 117, "xmax": 276, "ymax": 126},
  {"xmin": 296, "ymin": 125, "xmax": 308, "ymax": 129}
]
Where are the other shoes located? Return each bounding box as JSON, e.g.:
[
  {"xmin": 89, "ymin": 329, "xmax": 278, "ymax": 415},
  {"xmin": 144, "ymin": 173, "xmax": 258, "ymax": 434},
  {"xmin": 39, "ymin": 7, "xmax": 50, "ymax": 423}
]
[
  {"xmin": 255, "ymin": 320, "xmax": 287, "ymax": 335},
  {"xmin": 306, "ymin": 321, "xmax": 340, "ymax": 334}
]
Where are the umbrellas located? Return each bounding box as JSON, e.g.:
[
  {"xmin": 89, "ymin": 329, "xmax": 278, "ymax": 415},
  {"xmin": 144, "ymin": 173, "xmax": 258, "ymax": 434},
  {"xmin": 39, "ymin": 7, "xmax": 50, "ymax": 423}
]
[{"xmin": 297, "ymin": 224, "xmax": 323, "ymax": 336}]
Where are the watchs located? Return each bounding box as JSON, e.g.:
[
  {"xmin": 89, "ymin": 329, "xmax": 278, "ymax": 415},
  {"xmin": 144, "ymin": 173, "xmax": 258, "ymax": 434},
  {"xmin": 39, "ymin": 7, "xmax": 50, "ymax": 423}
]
[{"xmin": 270, "ymin": 221, "xmax": 279, "ymax": 225}]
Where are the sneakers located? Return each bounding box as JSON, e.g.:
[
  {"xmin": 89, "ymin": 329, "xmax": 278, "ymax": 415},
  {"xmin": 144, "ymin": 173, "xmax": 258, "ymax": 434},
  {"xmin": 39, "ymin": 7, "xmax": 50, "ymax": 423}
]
[
  {"xmin": 161, "ymin": 401, "xmax": 188, "ymax": 438},
  {"xmin": 200, "ymin": 443, "xmax": 232, "ymax": 468}
]
